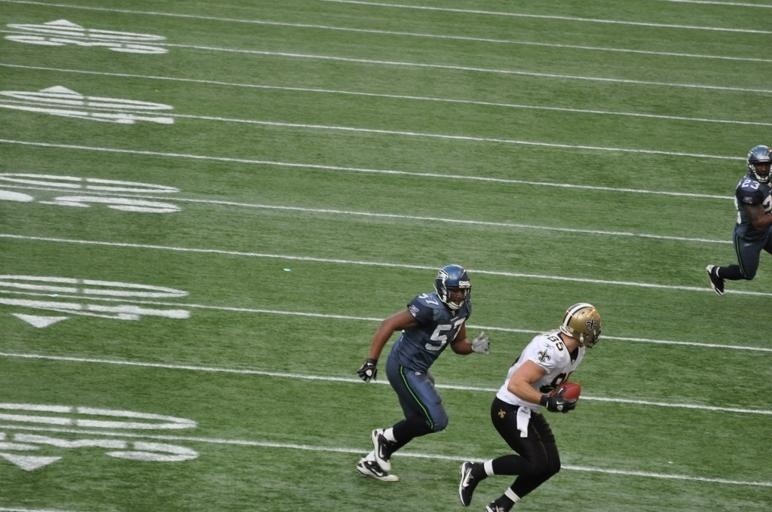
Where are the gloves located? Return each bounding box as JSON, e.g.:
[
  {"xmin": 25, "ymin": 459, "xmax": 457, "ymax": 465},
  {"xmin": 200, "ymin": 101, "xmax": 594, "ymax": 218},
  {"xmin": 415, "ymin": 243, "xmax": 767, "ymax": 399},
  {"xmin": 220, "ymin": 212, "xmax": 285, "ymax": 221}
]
[
  {"xmin": 471, "ymin": 331, "xmax": 491, "ymax": 355},
  {"xmin": 356, "ymin": 357, "xmax": 378, "ymax": 384},
  {"xmin": 539, "ymin": 386, "xmax": 577, "ymax": 414}
]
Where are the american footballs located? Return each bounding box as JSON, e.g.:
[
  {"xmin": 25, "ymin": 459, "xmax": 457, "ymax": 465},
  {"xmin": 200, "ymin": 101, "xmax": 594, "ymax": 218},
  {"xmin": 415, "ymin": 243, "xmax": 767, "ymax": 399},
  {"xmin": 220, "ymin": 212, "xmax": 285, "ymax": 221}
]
[{"xmin": 549, "ymin": 382, "xmax": 580, "ymax": 402}]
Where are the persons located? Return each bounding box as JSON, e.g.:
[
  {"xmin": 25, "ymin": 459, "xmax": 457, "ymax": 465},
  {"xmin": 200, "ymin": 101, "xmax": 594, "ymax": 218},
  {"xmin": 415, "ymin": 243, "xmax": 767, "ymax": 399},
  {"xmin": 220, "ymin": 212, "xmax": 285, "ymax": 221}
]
[
  {"xmin": 705, "ymin": 144, "xmax": 772, "ymax": 297},
  {"xmin": 459, "ymin": 303, "xmax": 601, "ymax": 512},
  {"xmin": 357, "ymin": 263, "xmax": 490, "ymax": 482}
]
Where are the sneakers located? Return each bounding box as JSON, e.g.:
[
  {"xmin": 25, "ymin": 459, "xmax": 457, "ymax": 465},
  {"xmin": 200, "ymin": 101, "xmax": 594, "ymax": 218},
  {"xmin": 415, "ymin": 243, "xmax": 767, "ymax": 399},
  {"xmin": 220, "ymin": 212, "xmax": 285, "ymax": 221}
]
[
  {"xmin": 371, "ymin": 428, "xmax": 400, "ymax": 472},
  {"xmin": 706, "ymin": 264, "xmax": 727, "ymax": 296},
  {"xmin": 485, "ymin": 501, "xmax": 509, "ymax": 512},
  {"xmin": 458, "ymin": 461, "xmax": 479, "ymax": 507},
  {"xmin": 356, "ymin": 457, "xmax": 398, "ymax": 482}
]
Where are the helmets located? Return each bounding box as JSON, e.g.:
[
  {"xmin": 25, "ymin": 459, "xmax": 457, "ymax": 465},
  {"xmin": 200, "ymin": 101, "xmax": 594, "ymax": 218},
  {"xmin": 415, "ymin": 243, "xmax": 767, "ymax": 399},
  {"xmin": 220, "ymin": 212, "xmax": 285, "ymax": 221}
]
[
  {"xmin": 745, "ymin": 144, "xmax": 772, "ymax": 183},
  {"xmin": 432, "ymin": 264, "xmax": 471, "ymax": 310},
  {"xmin": 558, "ymin": 302, "xmax": 602, "ymax": 349}
]
